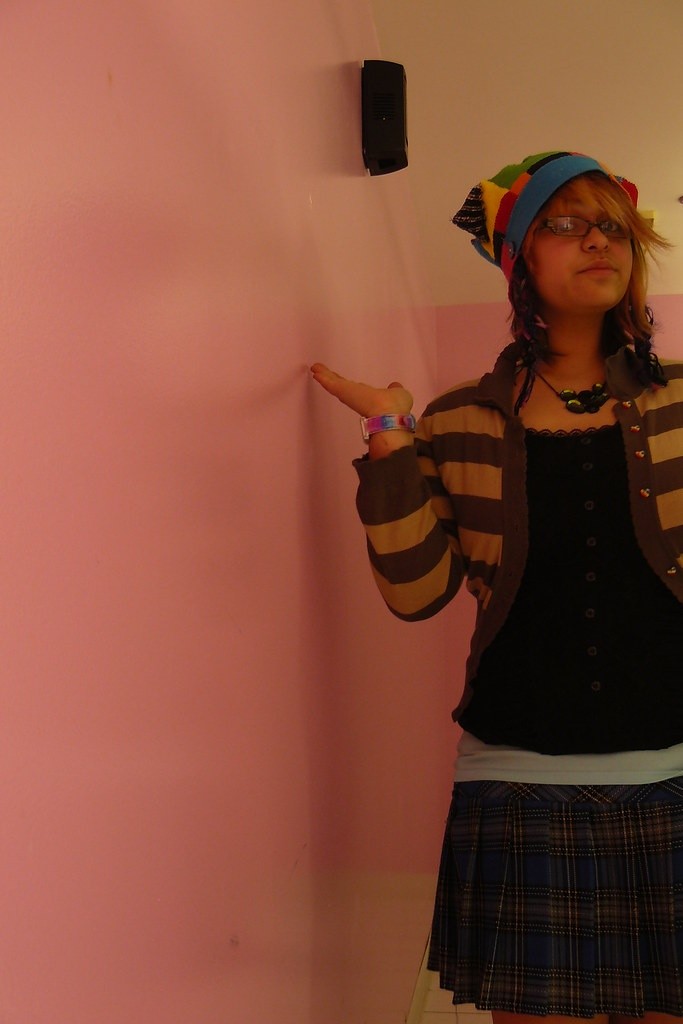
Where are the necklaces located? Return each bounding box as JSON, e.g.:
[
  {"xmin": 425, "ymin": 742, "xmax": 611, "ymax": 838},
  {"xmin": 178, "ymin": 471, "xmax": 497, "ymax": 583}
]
[{"xmin": 535, "ymin": 365, "xmax": 615, "ymax": 415}]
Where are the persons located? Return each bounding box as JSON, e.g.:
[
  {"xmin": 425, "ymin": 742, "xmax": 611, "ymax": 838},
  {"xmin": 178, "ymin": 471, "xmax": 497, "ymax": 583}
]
[{"xmin": 311, "ymin": 150, "xmax": 683, "ymax": 1024}]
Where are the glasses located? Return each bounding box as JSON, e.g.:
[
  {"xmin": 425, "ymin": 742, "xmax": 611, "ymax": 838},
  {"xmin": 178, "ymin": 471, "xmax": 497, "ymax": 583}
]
[{"xmin": 533, "ymin": 215, "xmax": 629, "ymax": 239}]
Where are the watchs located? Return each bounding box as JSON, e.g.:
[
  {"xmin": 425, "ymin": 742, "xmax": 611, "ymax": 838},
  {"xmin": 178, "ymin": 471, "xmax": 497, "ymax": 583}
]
[{"xmin": 360, "ymin": 414, "xmax": 415, "ymax": 443}]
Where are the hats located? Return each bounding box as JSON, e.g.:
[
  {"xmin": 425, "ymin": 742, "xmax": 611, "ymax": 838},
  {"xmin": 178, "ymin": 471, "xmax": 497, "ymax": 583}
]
[{"xmin": 452, "ymin": 151, "xmax": 638, "ymax": 285}]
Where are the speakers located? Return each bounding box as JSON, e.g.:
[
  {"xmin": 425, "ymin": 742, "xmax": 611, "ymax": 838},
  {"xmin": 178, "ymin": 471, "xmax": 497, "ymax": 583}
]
[{"xmin": 361, "ymin": 60, "xmax": 407, "ymax": 174}]
